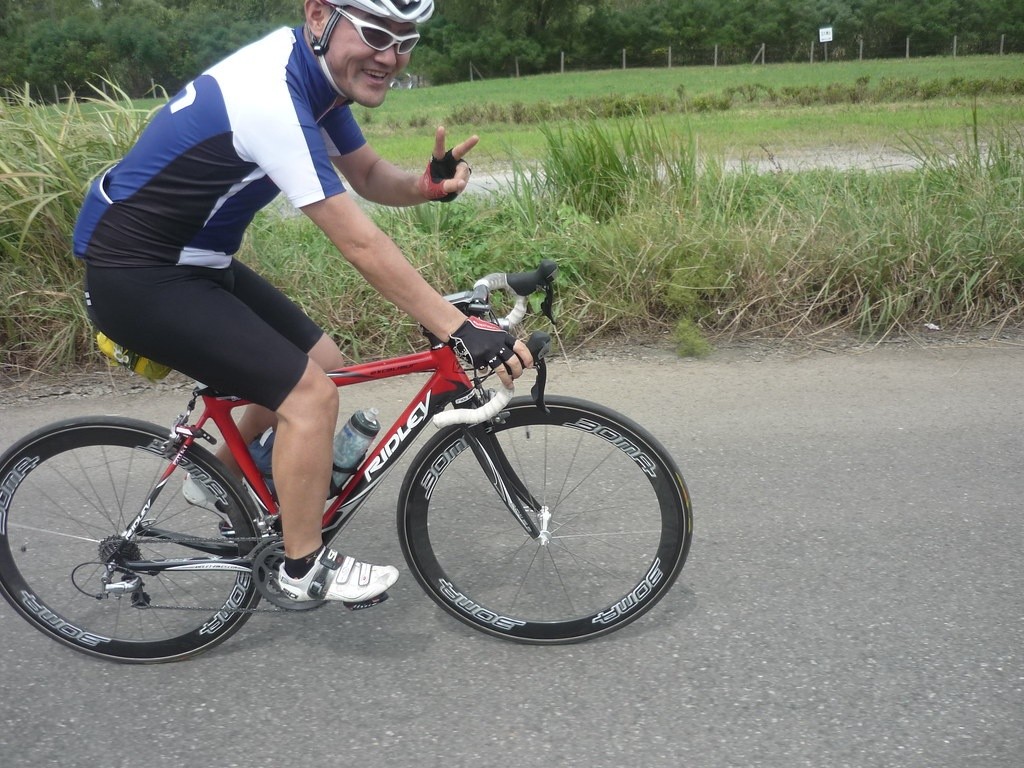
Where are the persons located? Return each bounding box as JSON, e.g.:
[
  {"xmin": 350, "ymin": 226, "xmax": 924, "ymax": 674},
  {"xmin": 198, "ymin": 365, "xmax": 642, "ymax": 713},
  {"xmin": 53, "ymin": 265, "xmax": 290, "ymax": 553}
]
[{"xmin": 72, "ymin": 0, "xmax": 533, "ymax": 603}]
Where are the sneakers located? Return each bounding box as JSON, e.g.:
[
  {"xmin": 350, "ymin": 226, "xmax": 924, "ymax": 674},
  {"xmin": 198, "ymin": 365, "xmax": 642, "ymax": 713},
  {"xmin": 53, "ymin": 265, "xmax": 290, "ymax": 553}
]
[
  {"xmin": 182, "ymin": 473, "xmax": 232, "ymax": 527},
  {"xmin": 278, "ymin": 546, "xmax": 400, "ymax": 602}
]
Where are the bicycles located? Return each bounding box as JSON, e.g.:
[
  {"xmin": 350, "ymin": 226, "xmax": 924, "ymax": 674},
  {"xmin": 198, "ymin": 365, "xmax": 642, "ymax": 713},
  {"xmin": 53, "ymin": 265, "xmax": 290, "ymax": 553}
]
[{"xmin": 0, "ymin": 259, "xmax": 693, "ymax": 665}]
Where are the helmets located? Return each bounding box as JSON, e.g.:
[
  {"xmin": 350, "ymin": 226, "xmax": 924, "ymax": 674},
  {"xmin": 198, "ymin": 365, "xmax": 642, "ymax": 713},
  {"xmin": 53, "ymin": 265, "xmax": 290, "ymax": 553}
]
[{"xmin": 326, "ymin": 0, "xmax": 435, "ymax": 25}]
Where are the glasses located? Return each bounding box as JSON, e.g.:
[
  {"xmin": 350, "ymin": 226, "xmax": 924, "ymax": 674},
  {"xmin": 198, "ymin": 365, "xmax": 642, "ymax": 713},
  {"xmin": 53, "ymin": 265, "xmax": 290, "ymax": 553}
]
[{"xmin": 321, "ymin": 0, "xmax": 420, "ymax": 54}]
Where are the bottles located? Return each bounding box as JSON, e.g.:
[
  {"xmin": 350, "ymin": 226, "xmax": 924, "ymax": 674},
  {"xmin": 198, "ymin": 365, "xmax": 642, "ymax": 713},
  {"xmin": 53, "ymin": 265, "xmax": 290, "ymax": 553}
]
[{"xmin": 326, "ymin": 408, "xmax": 381, "ymax": 500}]
